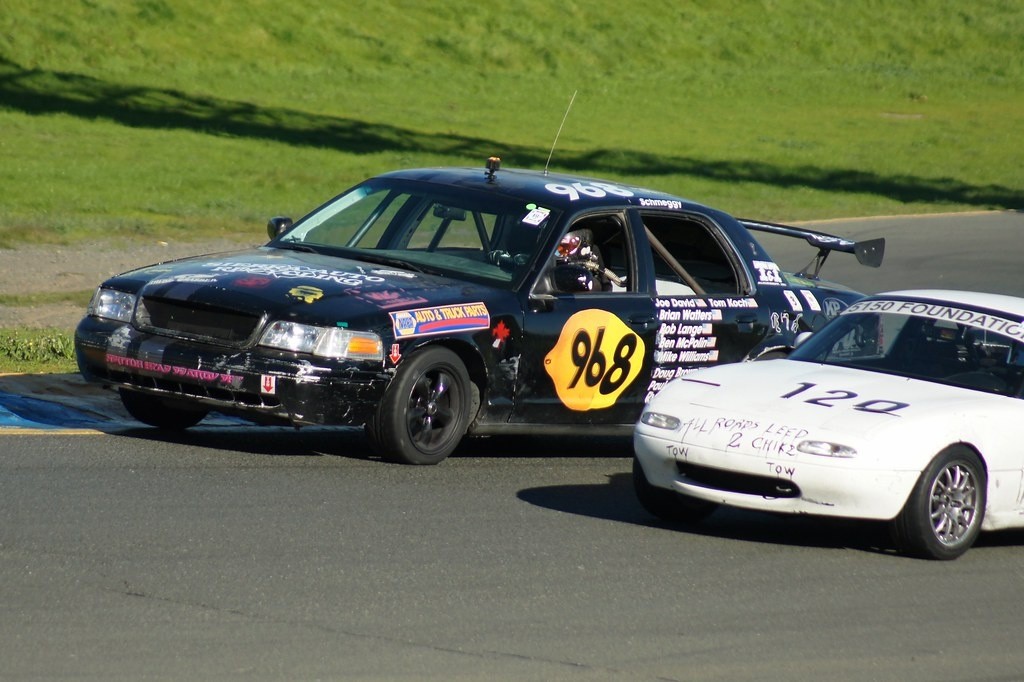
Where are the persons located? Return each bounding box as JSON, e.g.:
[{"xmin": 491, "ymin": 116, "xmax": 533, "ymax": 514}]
[{"xmin": 486, "ymin": 220, "xmax": 609, "ymax": 294}]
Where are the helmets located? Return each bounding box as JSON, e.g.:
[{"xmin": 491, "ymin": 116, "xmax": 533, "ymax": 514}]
[{"xmin": 538, "ymin": 217, "xmax": 594, "ymax": 262}]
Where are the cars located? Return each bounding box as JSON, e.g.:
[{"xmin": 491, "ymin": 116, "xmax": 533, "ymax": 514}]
[
  {"xmin": 73, "ymin": 154, "xmax": 885, "ymax": 468},
  {"xmin": 626, "ymin": 285, "xmax": 1023, "ymax": 563}
]
